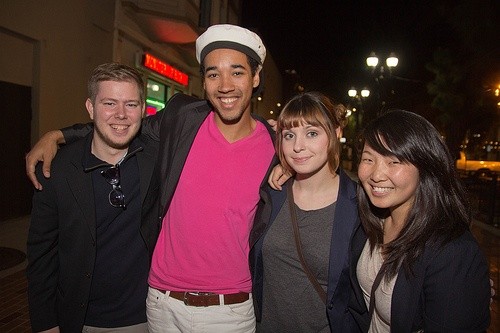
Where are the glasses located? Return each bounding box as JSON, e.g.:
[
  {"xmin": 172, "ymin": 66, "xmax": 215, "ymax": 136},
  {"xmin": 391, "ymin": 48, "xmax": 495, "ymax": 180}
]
[{"xmin": 105, "ymin": 163, "xmax": 127, "ymax": 210}]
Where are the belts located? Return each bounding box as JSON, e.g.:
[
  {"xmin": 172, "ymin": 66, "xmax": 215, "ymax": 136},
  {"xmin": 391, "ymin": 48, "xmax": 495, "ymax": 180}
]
[{"xmin": 158, "ymin": 290, "xmax": 249, "ymax": 306}]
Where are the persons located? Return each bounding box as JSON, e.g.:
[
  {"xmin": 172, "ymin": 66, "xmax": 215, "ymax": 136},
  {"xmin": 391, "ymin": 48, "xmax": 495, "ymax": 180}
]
[
  {"xmin": 248, "ymin": 92, "xmax": 495, "ymax": 333},
  {"xmin": 27, "ymin": 24, "xmax": 280, "ymax": 333},
  {"xmin": 268, "ymin": 110, "xmax": 490, "ymax": 333},
  {"xmin": 26, "ymin": 63, "xmax": 277, "ymax": 333}
]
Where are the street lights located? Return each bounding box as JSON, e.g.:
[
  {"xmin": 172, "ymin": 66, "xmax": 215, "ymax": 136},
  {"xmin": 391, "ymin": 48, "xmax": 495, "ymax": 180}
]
[
  {"xmin": 347, "ymin": 86, "xmax": 371, "ymax": 171},
  {"xmin": 365, "ymin": 51, "xmax": 399, "ymax": 118}
]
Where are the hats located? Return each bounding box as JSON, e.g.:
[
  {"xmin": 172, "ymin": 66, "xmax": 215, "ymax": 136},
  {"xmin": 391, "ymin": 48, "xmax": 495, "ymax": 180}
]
[{"xmin": 195, "ymin": 24, "xmax": 267, "ymax": 66}]
